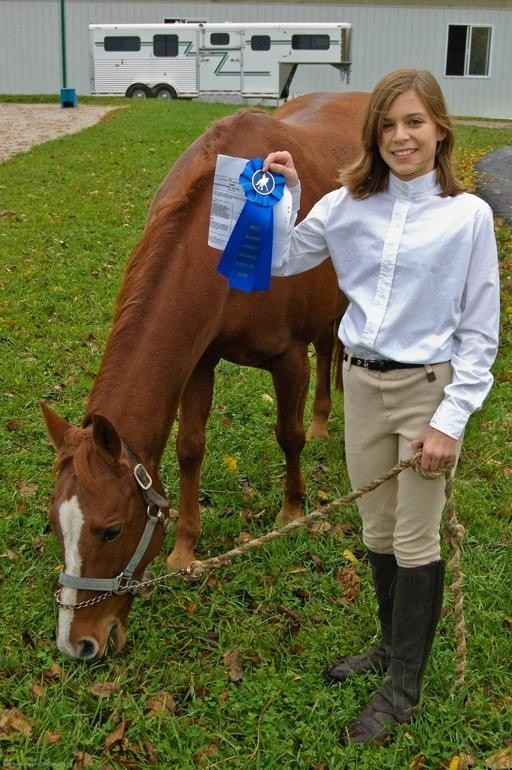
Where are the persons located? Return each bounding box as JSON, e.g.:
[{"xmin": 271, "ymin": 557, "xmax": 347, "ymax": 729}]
[{"xmin": 258, "ymin": 67, "xmax": 504, "ymax": 754}]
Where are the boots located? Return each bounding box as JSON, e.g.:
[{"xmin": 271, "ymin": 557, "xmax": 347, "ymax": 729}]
[
  {"xmin": 341, "ymin": 559, "xmax": 446, "ymax": 745},
  {"xmin": 324, "ymin": 550, "xmax": 398, "ymax": 684}
]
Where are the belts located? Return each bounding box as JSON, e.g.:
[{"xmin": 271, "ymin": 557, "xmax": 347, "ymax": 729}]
[{"xmin": 344, "ymin": 355, "xmax": 439, "ymax": 373}]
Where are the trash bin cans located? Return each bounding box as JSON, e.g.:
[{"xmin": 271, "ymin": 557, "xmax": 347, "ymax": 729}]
[{"xmin": 60, "ymin": 88, "xmax": 76, "ymax": 108}]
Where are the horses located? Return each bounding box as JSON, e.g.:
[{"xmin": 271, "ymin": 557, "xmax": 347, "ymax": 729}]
[{"xmin": 37, "ymin": 88, "xmax": 374, "ymax": 664}]
